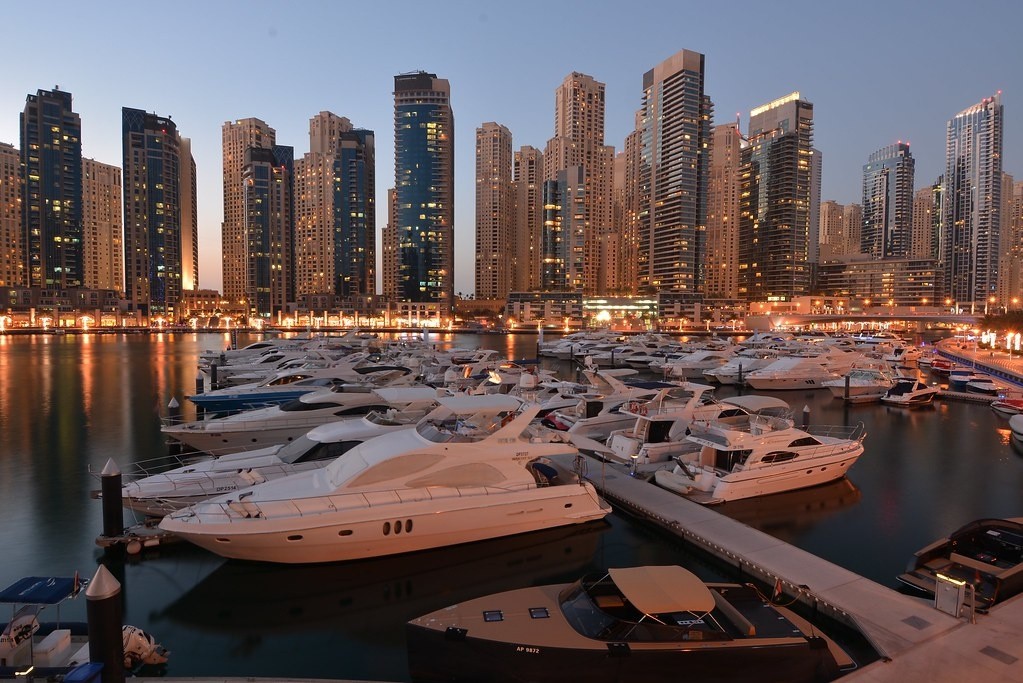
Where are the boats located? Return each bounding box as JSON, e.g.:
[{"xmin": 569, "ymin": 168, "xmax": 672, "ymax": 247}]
[
  {"xmin": 948, "ymin": 367, "xmax": 978, "ymax": 392},
  {"xmin": 820, "ymin": 369, "xmax": 895, "ymax": 404},
  {"xmin": 893, "ymin": 516, "xmax": 1023, "ymax": 616},
  {"xmin": 404, "ymin": 565, "xmax": 857, "ymax": 682},
  {"xmin": 966, "ymin": 377, "xmax": 999, "ymax": 397},
  {"xmin": 990, "ymin": 400, "xmax": 1023, "ymax": 422},
  {"xmin": 880, "ymin": 376, "xmax": 940, "ymax": 410},
  {"xmin": 161, "ymin": 398, "xmax": 615, "ymax": 566},
  {"xmin": 653, "ymin": 412, "xmax": 868, "ymax": 506},
  {"xmin": 90, "ymin": 329, "xmax": 960, "ymax": 519},
  {"xmin": 0, "ymin": 575, "xmax": 170, "ymax": 683},
  {"xmin": 1009, "ymin": 414, "xmax": 1023, "ymax": 453}
]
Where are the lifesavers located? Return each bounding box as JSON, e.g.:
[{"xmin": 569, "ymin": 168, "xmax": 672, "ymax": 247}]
[
  {"xmin": 629, "ymin": 402, "xmax": 638, "ymax": 413},
  {"xmin": 640, "ymin": 404, "xmax": 648, "ymax": 416},
  {"xmin": 502, "ymin": 416, "xmax": 514, "ymax": 427}
]
